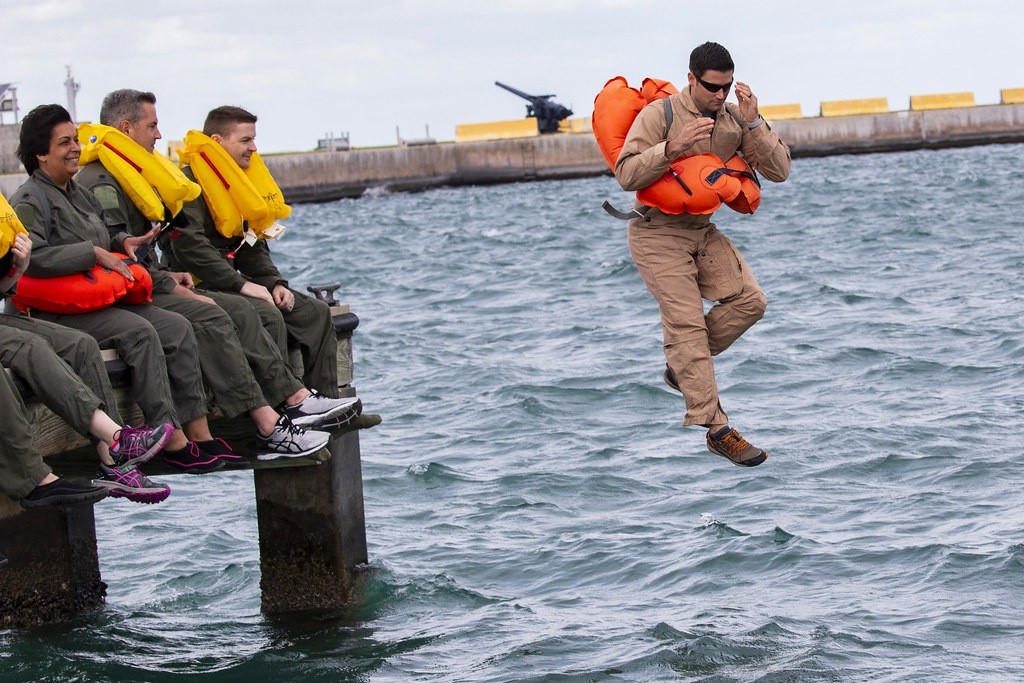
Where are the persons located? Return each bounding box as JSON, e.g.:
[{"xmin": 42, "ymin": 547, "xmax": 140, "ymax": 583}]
[
  {"xmin": 73, "ymin": 88, "xmax": 362, "ymax": 460},
  {"xmin": 9, "ymin": 103, "xmax": 250, "ymax": 474},
  {"xmin": 613, "ymin": 42, "xmax": 792, "ymax": 468},
  {"xmin": 157, "ymin": 104, "xmax": 382, "ymax": 462},
  {"xmin": 0, "ymin": 233, "xmax": 109, "ymax": 509},
  {"xmin": 0, "ymin": 312, "xmax": 174, "ymax": 504}
]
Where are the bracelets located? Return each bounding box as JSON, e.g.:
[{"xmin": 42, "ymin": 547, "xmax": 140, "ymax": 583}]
[{"xmin": 747, "ymin": 116, "xmax": 762, "ymax": 128}]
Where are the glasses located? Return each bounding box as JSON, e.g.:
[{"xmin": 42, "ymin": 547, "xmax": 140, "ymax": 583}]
[{"xmin": 692, "ymin": 71, "xmax": 734, "ymax": 93}]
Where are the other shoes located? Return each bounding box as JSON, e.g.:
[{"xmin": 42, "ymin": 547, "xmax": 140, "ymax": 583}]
[{"xmin": 333, "ymin": 413, "xmax": 382, "ymax": 440}]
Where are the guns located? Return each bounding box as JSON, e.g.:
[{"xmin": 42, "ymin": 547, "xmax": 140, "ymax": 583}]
[{"xmin": 495, "ymin": 80, "xmax": 572, "ymax": 134}]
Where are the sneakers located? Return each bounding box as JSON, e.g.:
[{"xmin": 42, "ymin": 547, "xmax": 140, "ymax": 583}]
[
  {"xmin": 283, "ymin": 389, "xmax": 363, "ymax": 435},
  {"xmin": 108, "ymin": 422, "xmax": 174, "ymax": 467},
  {"xmin": 190, "ymin": 438, "xmax": 251, "ymax": 465},
  {"xmin": 157, "ymin": 441, "xmax": 226, "ymax": 474},
  {"xmin": 255, "ymin": 414, "xmax": 331, "ymax": 460},
  {"xmin": 20, "ymin": 473, "xmax": 110, "ymax": 514},
  {"xmin": 664, "ymin": 368, "xmax": 681, "ymax": 392},
  {"xmin": 706, "ymin": 427, "xmax": 768, "ymax": 467},
  {"xmin": 90, "ymin": 464, "xmax": 170, "ymax": 504}
]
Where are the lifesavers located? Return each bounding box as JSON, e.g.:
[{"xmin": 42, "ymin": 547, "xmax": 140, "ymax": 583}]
[
  {"xmin": 74, "ymin": 123, "xmax": 202, "ymax": 221},
  {"xmin": 179, "ymin": 130, "xmax": 292, "ymax": 237},
  {"xmin": 8, "ymin": 252, "xmax": 155, "ymax": 311},
  {"xmin": 591, "ymin": 74, "xmax": 763, "ymax": 216}
]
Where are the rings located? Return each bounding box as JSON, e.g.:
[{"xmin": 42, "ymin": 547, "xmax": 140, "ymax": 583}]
[
  {"xmin": 749, "ymin": 94, "xmax": 752, "ymax": 98},
  {"xmin": 290, "ymin": 305, "xmax": 291, "ymax": 307}
]
